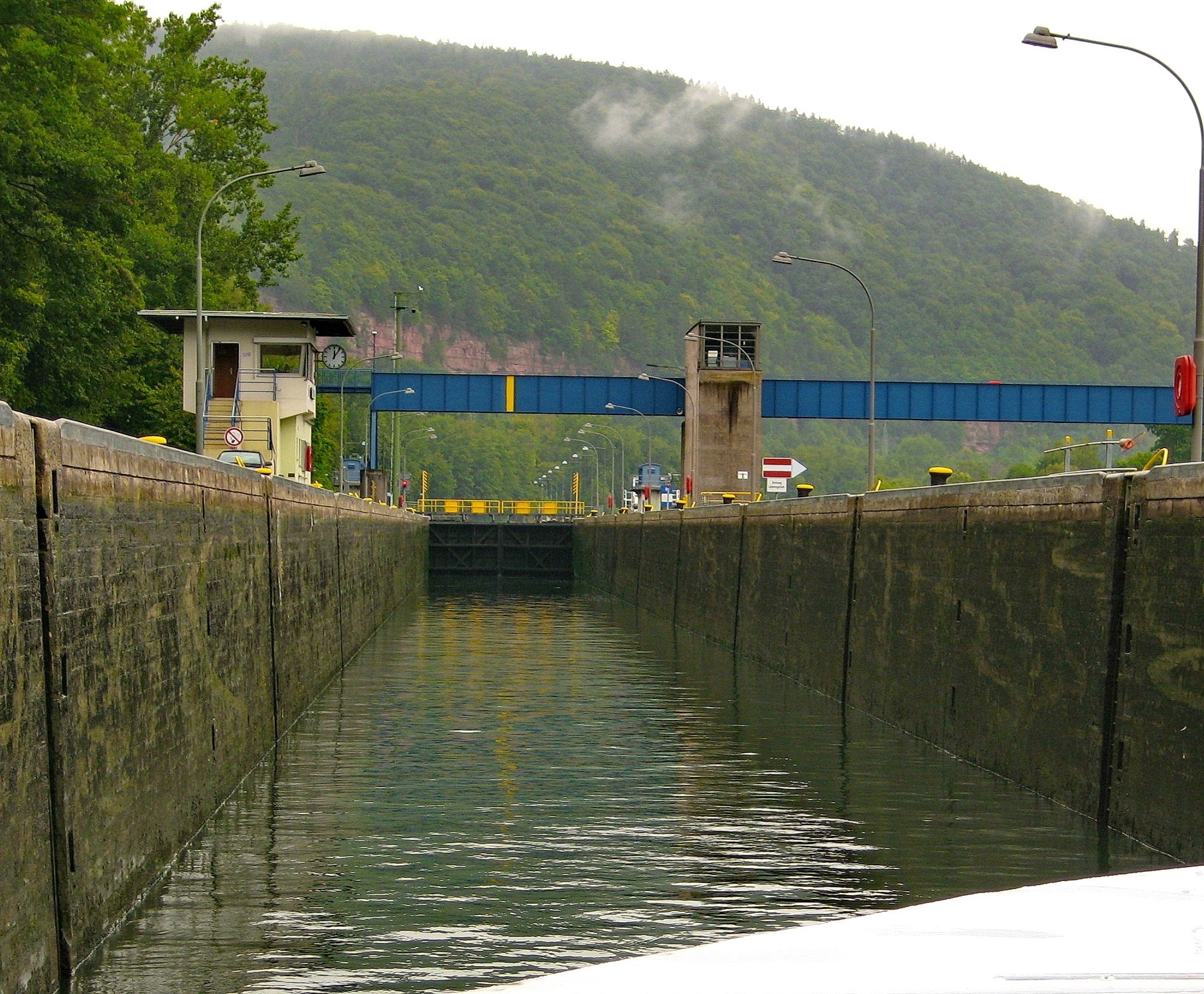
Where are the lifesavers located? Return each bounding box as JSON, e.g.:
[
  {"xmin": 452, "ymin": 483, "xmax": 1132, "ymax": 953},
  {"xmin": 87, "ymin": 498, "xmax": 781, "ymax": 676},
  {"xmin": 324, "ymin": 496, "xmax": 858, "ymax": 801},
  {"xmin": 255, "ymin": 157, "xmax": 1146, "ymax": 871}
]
[
  {"xmin": 306, "ymin": 445, "xmax": 312, "ymax": 472},
  {"xmin": 608, "ymin": 495, "xmax": 612, "ymax": 507},
  {"xmin": 400, "ymin": 495, "xmax": 403, "ymax": 507},
  {"xmin": 686, "ymin": 475, "xmax": 692, "ymax": 493},
  {"xmin": 644, "ymin": 485, "xmax": 648, "ymax": 499},
  {"xmin": 1175, "ymin": 366, "xmax": 1183, "ymax": 402}
]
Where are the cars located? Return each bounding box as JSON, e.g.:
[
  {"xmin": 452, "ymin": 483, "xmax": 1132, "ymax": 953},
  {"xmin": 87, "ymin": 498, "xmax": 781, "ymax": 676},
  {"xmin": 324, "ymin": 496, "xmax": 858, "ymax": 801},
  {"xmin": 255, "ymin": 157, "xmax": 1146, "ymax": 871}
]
[{"xmin": 216, "ymin": 450, "xmax": 274, "ymax": 475}]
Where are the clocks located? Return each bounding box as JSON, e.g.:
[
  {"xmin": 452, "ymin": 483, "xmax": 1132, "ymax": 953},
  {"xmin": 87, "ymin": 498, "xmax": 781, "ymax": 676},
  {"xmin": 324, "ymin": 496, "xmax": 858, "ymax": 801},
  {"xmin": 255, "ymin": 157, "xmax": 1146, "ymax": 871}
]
[{"xmin": 322, "ymin": 344, "xmax": 346, "ymax": 369}]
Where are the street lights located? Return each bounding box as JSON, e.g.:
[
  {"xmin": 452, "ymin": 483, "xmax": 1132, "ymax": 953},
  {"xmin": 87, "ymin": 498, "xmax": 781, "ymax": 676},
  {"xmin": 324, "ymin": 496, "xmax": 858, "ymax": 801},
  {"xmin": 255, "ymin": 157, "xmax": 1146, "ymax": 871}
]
[
  {"xmin": 604, "ymin": 401, "xmax": 652, "ymax": 506},
  {"xmin": 533, "ymin": 421, "xmax": 625, "ymax": 516},
  {"xmin": 340, "ymin": 352, "xmax": 404, "ymax": 494},
  {"xmin": 768, "ymin": 251, "xmax": 876, "ymax": 491},
  {"xmin": 361, "ymin": 386, "xmax": 437, "ymax": 492},
  {"xmin": 637, "ymin": 372, "xmax": 696, "ymax": 507},
  {"xmin": 195, "ymin": 159, "xmax": 328, "ymax": 455},
  {"xmin": 683, "ymin": 332, "xmax": 756, "ymax": 503},
  {"xmin": 1021, "ymin": 24, "xmax": 1204, "ymax": 463}
]
